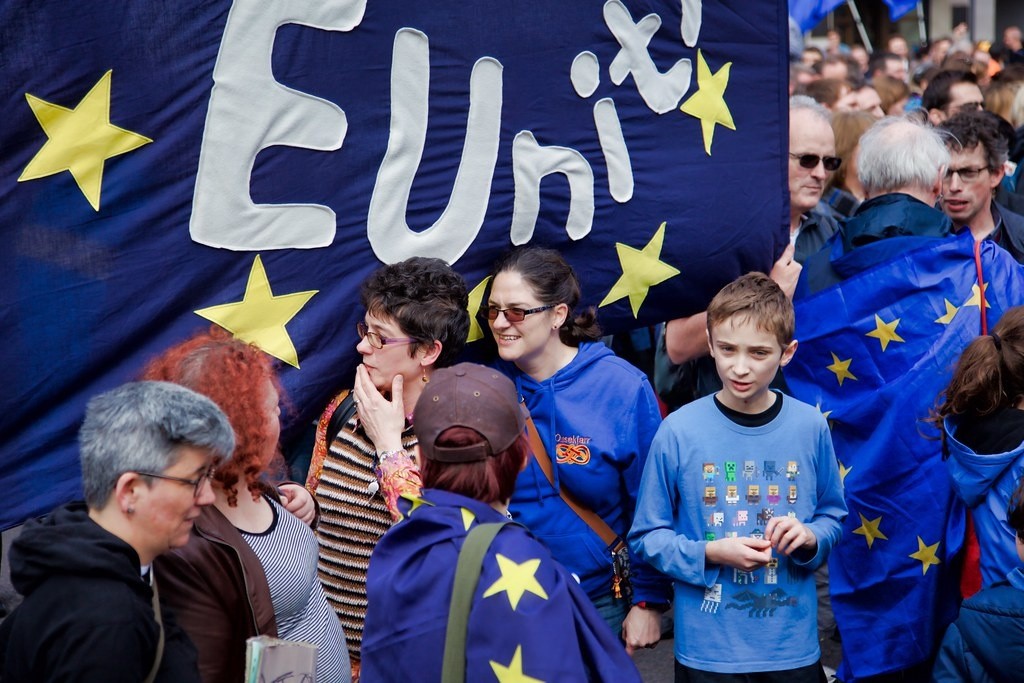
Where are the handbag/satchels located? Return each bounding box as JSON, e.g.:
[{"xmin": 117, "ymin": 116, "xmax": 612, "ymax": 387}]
[
  {"xmin": 961, "ymin": 510, "xmax": 980, "ymax": 598},
  {"xmin": 615, "ymin": 543, "xmax": 675, "ymax": 610}
]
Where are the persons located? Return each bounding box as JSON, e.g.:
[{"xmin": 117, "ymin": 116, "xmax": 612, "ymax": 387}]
[
  {"xmin": 156, "ymin": 22, "xmax": 1024, "ymax": 683},
  {"xmin": 0, "ymin": 380, "xmax": 235, "ymax": 683}
]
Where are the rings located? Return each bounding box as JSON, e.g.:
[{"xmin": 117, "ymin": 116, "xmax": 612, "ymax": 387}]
[{"xmin": 354, "ymin": 400, "xmax": 360, "ymax": 407}]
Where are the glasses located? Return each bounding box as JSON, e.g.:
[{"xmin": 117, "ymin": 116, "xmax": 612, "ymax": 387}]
[
  {"xmin": 788, "ymin": 153, "xmax": 842, "ymax": 172},
  {"xmin": 114, "ymin": 466, "xmax": 215, "ymax": 497},
  {"xmin": 940, "ymin": 102, "xmax": 986, "ymax": 112},
  {"xmin": 357, "ymin": 322, "xmax": 424, "ymax": 349},
  {"xmin": 481, "ymin": 304, "xmax": 558, "ymax": 322},
  {"xmin": 944, "ymin": 165, "xmax": 991, "ymax": 182}
]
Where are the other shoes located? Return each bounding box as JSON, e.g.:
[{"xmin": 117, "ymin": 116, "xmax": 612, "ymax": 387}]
[{"xmin": 658, "ymin": 616, "xmax": 674, "ymax": 634}]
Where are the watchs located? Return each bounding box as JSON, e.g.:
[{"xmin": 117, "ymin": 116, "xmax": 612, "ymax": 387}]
[
  {"xmin": 380, "ymin": 451, "xmax": 393, "ymax": 465},
  {"xmin": 637, "ymin": 602, "xmax": 668, "ymax": 611}
]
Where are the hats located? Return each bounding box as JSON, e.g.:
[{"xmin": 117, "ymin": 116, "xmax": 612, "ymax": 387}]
[{"xmin": 412, "ymin": 363, "xmax": 525, "ymax": 463}]
